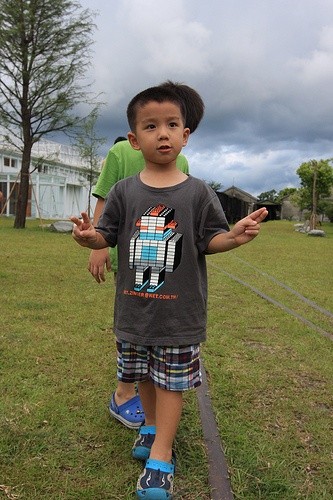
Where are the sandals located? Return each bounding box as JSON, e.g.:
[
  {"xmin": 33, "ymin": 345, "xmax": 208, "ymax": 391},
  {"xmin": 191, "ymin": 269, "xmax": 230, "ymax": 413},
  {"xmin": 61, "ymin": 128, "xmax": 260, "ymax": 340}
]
[
  {"xmin": 136, "ymin": 447, "xmax": 177, "ymax": 500},
  {"xmin": 108, "ymin": 391, "xmax": 149, "ymax": 429},
  {"xmin": 131, "ymin": 425, "xmax": 156, "ymax": 459}
]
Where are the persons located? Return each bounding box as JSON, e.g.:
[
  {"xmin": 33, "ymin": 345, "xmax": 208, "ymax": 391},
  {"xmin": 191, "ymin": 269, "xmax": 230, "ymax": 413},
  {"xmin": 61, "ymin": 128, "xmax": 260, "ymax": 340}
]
[
  {"xmin": 70, "ymin": 87, "xmax": 268, "ymax": 500},
  {"xmin": 88, "ymin": 81, "xmax": 205, "ymax": 432}
]
